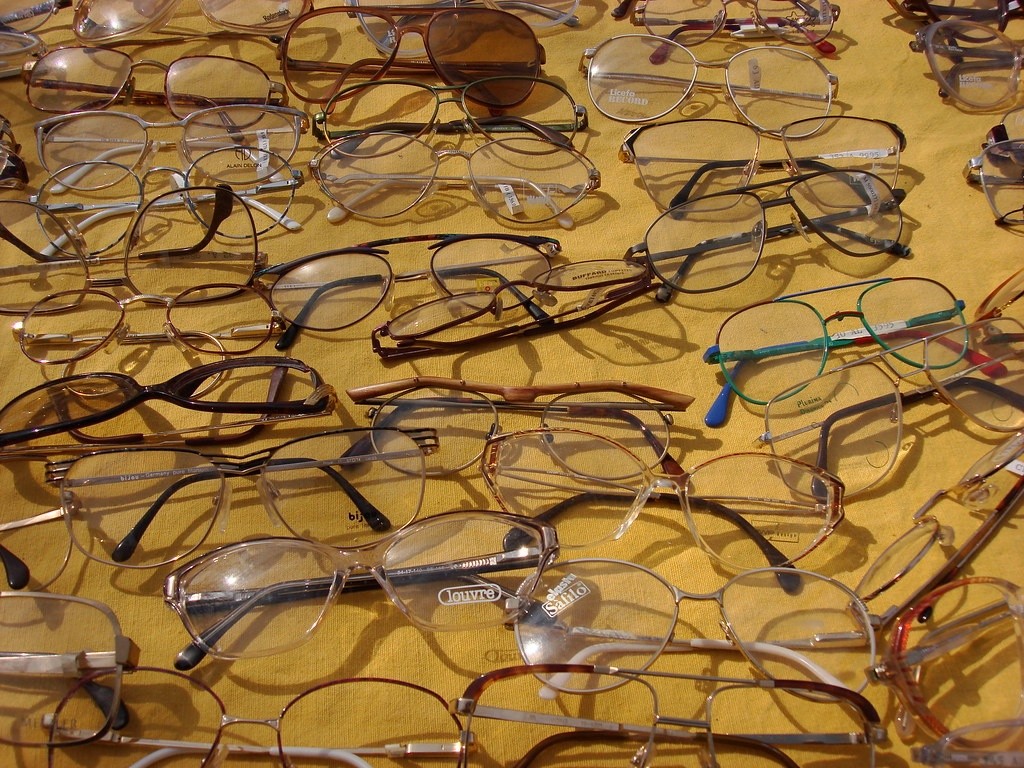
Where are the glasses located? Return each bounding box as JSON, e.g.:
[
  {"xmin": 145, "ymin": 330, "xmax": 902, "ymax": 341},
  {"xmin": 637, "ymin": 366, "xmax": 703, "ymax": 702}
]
[{"xmin": 1, "ymin": 1, "xmax": 1024, "ymax": 768}]
